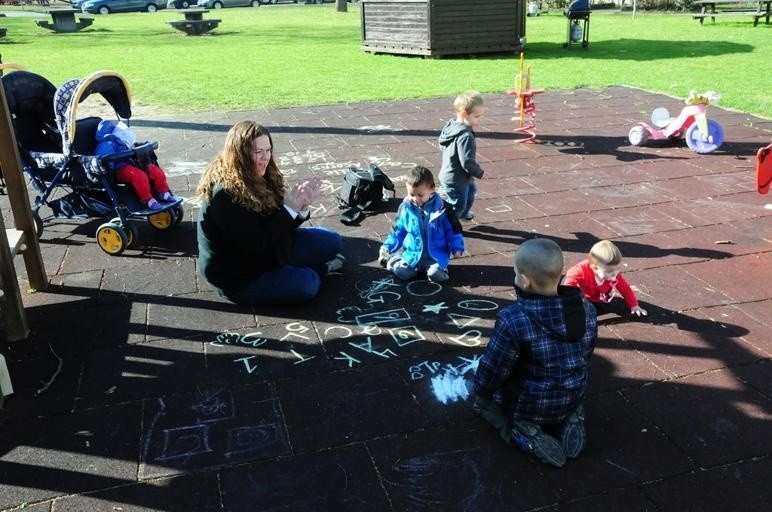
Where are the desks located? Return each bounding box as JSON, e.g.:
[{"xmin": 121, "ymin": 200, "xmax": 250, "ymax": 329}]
[
  {"xmin": 693, "ymin": 0, "xmax": 770, "ymax": 27},
  {"xmin": 563, "ymin": 0, "xmax": 591, "ymax": 48},
  {"xmin": 179, "ymin": 9, "xmax": 209, "ymax": 22},
  {"xmin": 46, "ymin": 9, "xmax": 82, "ymax": 25}
]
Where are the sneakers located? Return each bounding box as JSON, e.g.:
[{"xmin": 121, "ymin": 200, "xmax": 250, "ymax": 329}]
[
  {"xmin": 463, "ymin": 211, "xmax": 475, "ymax": 222},
  {"xmin": 379, "ymin": 252, "xmax": 399, "ymax": 268},
  {"xmin": 559, "ymin": 405, "xmax": 587, "ymax": 459},
  {"xmin": 325, "ymin": 254, "xmax": 347, "ymax": 276},
  {"xmin": 147, "ymin": 198, "xmax": 162, "ymax": 210},
  {"xmin": 160, "ymin": 191, "xmax": 177, "ymax": 202},
  {"xmin": 511, "ymin": 418, "xmax": 566, "ymax": 468}
]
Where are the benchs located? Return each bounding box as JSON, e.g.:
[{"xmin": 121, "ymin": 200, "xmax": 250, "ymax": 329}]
[
  {"xmin": 165, "ymin": 18, "xmax": 221, "ymax": 36},
  {"xmin": 35, "ymin": 18, "xmax": 95, "ymax": 33},
  {"xmin": 692, "ymin": 8, "xmax": 772, "ymax": 26}
]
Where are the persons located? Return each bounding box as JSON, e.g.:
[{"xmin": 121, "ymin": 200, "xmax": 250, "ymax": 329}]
[
  {"xmin": 94, "ymin": 117, "xmax": 177, "ymax": 211},
  {"xmin": 560, "ymin": 239, "xmax": 649, "ymax": 317},
  {"xmin": 435, "ymin": 89, "xmax": 490, "ymax": 218},
  {"xmin": 469, "ymin": 238, "xmax": 598, "ymax": 469},
  {"xmin": 196, "ymin": 118, "xmax": 346, "ymax": 307},
  {"xmin": 377, "ymin": 165, "xmax": 464, "ymax": 282}
]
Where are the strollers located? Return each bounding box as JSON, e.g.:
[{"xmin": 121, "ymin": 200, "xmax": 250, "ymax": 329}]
[{"xmin": 0, "ymin": 62, "xmax": 185, "ymax": 255}]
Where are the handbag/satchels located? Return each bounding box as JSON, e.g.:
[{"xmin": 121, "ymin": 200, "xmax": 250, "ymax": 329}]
[{"xmin": 337, "ymin": 162, "xmax": 395, "ymax": 226}]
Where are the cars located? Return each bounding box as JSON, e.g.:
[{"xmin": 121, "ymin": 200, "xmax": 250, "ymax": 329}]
[
  {"xmin": 197, "ymin": 0, "xmax": 264, "ymax": 10},
  {"xmin": 81, "ymin": 0, "xmax": 168, "ymax": 16}
]
